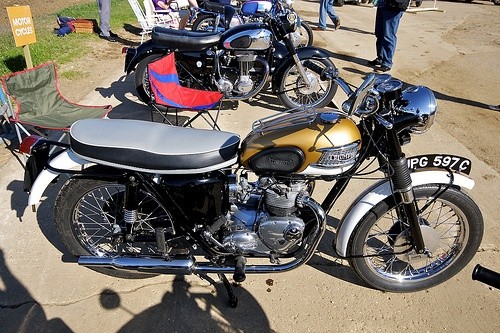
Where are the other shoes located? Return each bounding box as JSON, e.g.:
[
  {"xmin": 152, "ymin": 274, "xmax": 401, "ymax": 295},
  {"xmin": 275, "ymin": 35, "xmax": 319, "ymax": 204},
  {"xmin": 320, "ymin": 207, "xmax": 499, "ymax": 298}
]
[
  {"xmin": 312, "ymin": 27, "xmax": 323, "ymax": 31},
  {"xmin": 109, "ymin": 31, "xmax": 118, "ymax": 38},
  {"xmin": 99, "ymin": 35, "xmax": 116, "ymax": 42},
  {"xmin": 376, "ymin": 66, "xmax": 391, "ymax": 72},
  {"xmin": 333, "ymin": 19, "xmax": 341, "ymax": 30},
  {"xmin": 369, "ymin": 59, "xmax": 380, "ymax": 66}
]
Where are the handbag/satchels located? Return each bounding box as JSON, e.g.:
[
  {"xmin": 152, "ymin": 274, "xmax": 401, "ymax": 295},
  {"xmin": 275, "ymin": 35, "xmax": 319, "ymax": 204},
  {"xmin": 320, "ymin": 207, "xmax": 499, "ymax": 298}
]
[{"xmin": 54, "ymin": 14, "xmax": 76, "ymax": 37}]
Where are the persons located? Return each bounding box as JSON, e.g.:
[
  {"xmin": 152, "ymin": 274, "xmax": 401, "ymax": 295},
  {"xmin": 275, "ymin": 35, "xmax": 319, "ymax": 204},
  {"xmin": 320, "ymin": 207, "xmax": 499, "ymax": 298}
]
[
  {"xmin": 367, "ymin": 0, "xmax": 410, "ymax": 72},
  {"xmin": 96, "ymin": 0, "xmax": 118, "ymax": 41},
  {"xmin": 310, "ymin": 0, "xmax": 343, "ymax": 31},
  {"xmin": 152, "ymin": 0, "xmax": 190, "ymax": 31}
]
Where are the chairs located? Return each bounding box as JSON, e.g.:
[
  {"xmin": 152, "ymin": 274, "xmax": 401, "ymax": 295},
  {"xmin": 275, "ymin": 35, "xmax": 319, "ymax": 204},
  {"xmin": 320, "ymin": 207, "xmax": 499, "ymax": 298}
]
[
  {"xmin": 127, "ymin": 0, "xmax": 200, "ymax": 45},
  {"xmin": 1, "ymin": 60, "xmax": 113, "ymax": 196},
  {"xmin": 146, "ymin": 52, "xmax": 225, "ymax": 132}
]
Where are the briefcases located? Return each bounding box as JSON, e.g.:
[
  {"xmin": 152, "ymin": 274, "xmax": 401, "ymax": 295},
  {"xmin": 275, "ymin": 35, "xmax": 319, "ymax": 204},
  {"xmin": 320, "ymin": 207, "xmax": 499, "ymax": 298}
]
[{"xmin": 72, "ymin": 18, "xmax": 97, "ymax": 34}]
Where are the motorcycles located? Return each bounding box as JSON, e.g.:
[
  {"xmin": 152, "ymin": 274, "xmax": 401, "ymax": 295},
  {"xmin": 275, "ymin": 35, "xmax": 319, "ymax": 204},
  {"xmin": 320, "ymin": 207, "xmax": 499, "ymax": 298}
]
[
  {"xmin": 22, "ymin": 59, "xmax": 486, "ymax": 309},
  {"xmin": 120, "ymin": 0, "xmax": 341, "ymax": 113}
]
